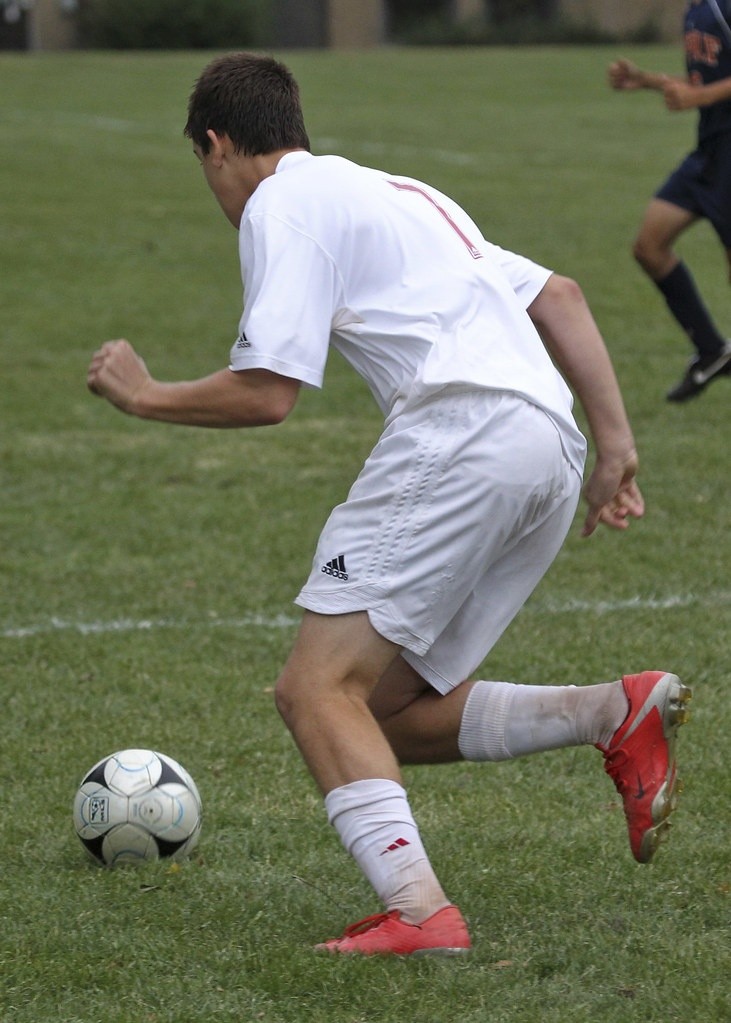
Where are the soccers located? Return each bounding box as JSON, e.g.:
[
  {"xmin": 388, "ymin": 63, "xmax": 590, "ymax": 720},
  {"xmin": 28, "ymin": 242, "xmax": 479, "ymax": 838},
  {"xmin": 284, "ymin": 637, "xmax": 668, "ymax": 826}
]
[{"xmin": 72, "ymin": 748, "xmax": 206, "ymax": 868}]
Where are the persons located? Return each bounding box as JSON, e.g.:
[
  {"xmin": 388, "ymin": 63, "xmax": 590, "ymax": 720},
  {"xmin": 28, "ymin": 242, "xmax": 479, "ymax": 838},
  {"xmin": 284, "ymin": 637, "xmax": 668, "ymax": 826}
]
[
  {"xmin": 84, "ymin": 54, "xmax": 694, "ymax": 954},
  {"xmin": 607, "ymin": 0, "xmax": 731, "ymax": 406}
]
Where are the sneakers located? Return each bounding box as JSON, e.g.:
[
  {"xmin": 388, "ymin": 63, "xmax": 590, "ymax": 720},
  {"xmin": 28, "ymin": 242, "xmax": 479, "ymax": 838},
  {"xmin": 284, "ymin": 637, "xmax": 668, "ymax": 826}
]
[
  {"xmin": 668, "ymin": 340, "xmax": 731, "ymax": 403},
  {"xmin": 593, "ymin": 670, "xmax": 693, "ymax": 865},
  {"xmin": 314, "ymin": 904, "xmax": 470, "ymax": 962}
]
